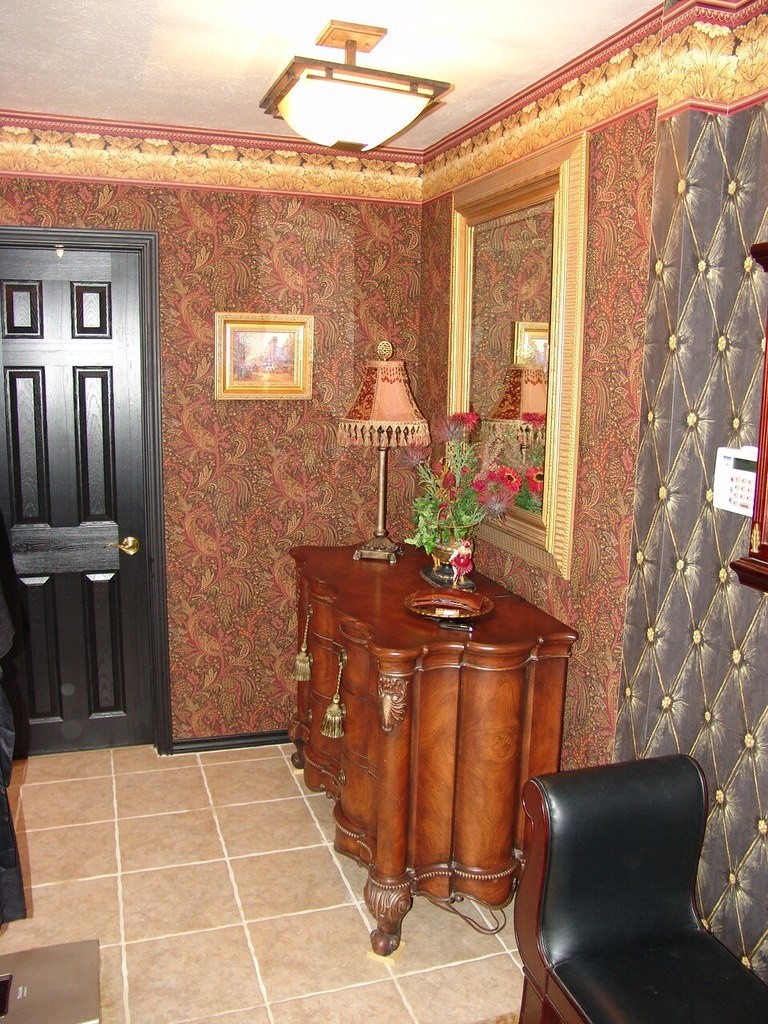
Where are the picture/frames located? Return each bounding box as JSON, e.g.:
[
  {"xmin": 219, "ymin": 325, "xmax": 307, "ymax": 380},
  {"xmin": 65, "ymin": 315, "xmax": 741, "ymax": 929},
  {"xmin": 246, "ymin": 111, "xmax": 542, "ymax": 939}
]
[
  {"xmin": 513, "ymin": 321, "xmax": 549, "ymax": 375},
  {"xmin": 214, "ymin": 311, "xmax": 315, "ymax": 400}
]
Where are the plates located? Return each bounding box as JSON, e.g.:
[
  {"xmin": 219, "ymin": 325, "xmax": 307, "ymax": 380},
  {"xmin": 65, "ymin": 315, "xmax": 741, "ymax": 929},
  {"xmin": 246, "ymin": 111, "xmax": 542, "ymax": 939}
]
[{"xmin": 404, "ymin": 589, "xmax": 495, "ymax": 622}]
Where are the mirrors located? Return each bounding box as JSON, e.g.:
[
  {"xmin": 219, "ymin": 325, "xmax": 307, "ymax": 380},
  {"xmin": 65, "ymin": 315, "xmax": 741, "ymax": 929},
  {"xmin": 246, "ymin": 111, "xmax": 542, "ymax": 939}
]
[{"xmin": 439, "ymin": 131, "xmax": 593, "ymax": 581}]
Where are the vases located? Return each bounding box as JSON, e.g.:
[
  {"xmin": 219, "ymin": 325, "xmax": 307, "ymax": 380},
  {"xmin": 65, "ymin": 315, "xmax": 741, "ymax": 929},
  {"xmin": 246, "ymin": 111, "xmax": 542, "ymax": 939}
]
[{"xmin": 421, "ymin": 523, "xmax": 477, "ymax": 590}]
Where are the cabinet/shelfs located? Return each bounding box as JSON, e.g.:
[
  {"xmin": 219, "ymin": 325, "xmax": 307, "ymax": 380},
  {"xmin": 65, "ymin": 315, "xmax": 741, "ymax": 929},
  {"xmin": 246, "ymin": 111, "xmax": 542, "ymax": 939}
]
[{"xmin": 287, "ymin": 542, "xmax": 578, "ymax": 955}]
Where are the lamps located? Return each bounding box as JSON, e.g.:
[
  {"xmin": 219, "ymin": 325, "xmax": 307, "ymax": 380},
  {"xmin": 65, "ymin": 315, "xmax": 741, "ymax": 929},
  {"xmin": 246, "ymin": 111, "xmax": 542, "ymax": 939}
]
[
  {"xmin": 258, "ymin": 20, "xmax": 451, "ymax": 152},
  {"xmin": 335, "ymin": 341, "xmax": 431, "ymax": 566},
  {"xmin": 485, "ymin": 345, "xmax": 547, "ymax": 421}
]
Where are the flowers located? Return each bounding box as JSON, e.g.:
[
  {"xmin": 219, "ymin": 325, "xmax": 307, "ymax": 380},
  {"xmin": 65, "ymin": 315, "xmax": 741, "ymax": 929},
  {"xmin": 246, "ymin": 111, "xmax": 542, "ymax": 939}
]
[
  {"xmin": 406, "ymin": 412, "xmax": 524, "ymax": 555},
  {"xmin": 484, "ymin": 411, "xmax": 545, "ymax": 512}
]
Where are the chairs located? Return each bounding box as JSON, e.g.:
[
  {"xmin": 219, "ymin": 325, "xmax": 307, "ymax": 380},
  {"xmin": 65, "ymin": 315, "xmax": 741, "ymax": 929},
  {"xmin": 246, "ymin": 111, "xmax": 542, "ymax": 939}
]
[{"xmin": 513, "ymin": 754, "xmax": 768, "ymax": 1024}]
